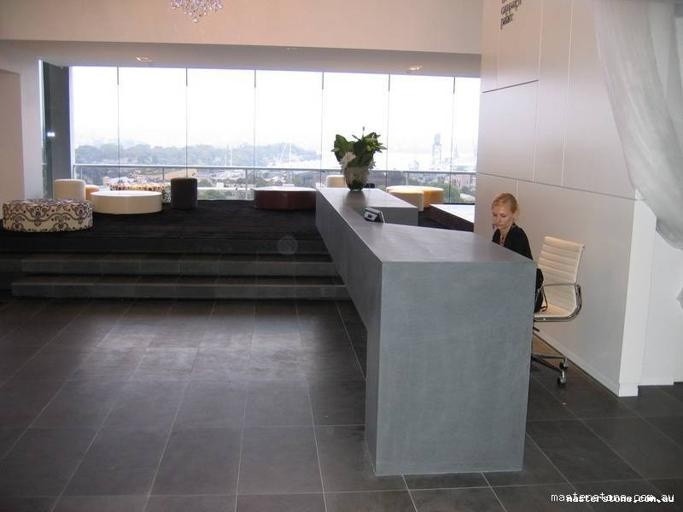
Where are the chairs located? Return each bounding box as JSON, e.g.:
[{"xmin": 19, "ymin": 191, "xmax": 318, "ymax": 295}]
[{"xmin": 531, "ymin": 235, "xmax": 585, "ymax": 387}]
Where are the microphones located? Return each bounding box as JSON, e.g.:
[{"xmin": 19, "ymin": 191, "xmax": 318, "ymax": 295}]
[{"xmin": 492, "ymin": 223, "xmax": 495, "ymax": 230}]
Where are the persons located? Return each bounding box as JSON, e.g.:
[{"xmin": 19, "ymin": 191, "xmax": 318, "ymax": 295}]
[{"xmin": 490, "ymin": 191, "xmax": 545, "ymax": 315}]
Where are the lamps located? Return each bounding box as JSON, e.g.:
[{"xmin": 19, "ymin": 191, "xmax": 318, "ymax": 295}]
[{"xmin": 170, "ymin": 0, "xmax": 224, "ymax": 24}]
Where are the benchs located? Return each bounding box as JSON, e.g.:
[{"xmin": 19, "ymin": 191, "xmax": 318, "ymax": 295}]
[
  {"xmin": 251, "ymin": 187, "xmax": 316, "ymax": 210},
  {"xmin": 110, "ymin": 182, "xmax": 171, "ymax": 203},
  {"xmin": 2, "ymin": 198, "xmax": 94, "ymax": 233}
]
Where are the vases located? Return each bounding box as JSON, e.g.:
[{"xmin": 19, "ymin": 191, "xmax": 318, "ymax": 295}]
[{"xmin": 345, "ymin": 165, "xmax": 369, "ymax": 191}]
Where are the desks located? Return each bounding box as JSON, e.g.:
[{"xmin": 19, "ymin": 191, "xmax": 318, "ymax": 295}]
[
  {"xmin": 326, "ymin": 175, "xmax": 346, "ymax": 188},
  {"xmin": 53, "ymin": 178, "xmax": 86, "ymax": 201},
  {"xmin": 171, "ymin": 177, "xmax": 197, "ymax": 210},
  {"xmin": 85, "ymin": 186, "xmax": 99, "ymax": 201}
]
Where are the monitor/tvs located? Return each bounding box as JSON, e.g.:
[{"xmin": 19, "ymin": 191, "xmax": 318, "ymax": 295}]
[{"xmin": 363, "ymin": 207, "xmax": 386, "ymax": 223}]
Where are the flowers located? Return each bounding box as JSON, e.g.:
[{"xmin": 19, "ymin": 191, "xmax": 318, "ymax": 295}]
[{"xmin": 331, "ymin": 126, "xmax": 388, "ymax": 174}]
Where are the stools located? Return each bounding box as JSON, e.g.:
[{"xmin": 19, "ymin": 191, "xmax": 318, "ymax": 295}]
[
  {"xmin": 388, "ymin": 189, "xmax": 424, "ymax": 212},
  {"xmin": 90, "ymin": 190, "xmax": 163, "ymax": 215},
  {"xmin": 386, "ymin": 185, "xmax": 445, "ymax": 208}
]
